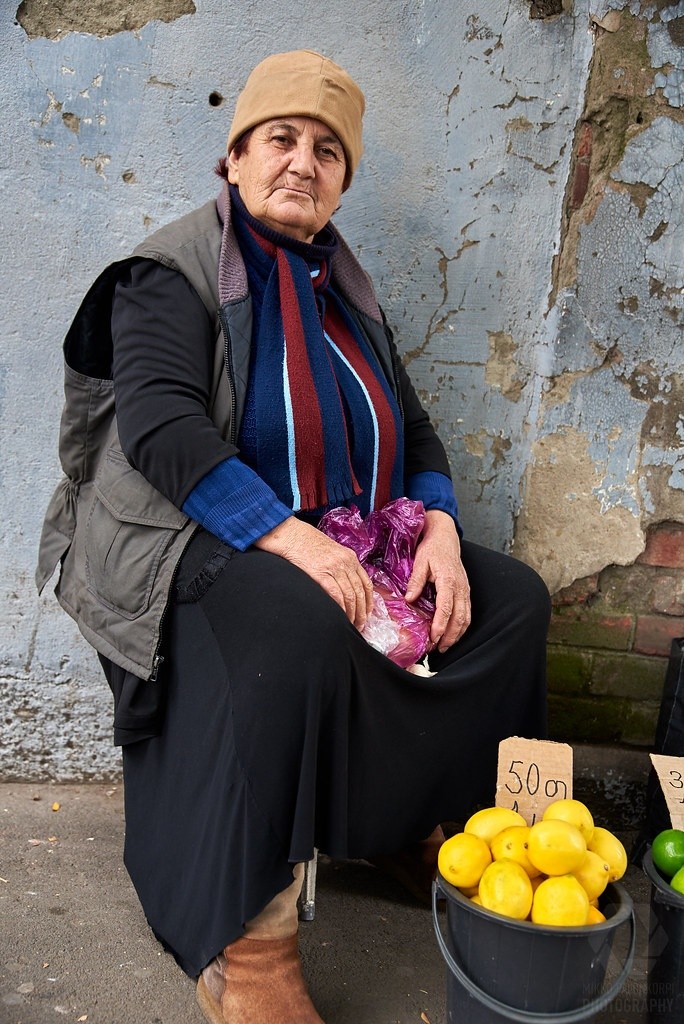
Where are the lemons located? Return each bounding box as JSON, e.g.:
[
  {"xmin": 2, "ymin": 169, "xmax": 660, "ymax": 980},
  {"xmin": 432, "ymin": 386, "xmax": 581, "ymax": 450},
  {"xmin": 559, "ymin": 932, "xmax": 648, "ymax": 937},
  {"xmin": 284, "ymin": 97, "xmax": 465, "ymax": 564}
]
[
  {"xmin": 651, "ymin": 828, "xmax": 684, "ymax": 894},
  {"xmin": 437, "ymin": 799, "xmax": 627, "ymax": 926}
]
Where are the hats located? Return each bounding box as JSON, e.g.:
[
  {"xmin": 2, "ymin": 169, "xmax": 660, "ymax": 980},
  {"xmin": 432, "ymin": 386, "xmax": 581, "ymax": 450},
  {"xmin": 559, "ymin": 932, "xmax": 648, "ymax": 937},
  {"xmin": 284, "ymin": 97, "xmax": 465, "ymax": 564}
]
[{"xmin": 227, "ymin": 50, "xmax": 365, "ymax": 194}]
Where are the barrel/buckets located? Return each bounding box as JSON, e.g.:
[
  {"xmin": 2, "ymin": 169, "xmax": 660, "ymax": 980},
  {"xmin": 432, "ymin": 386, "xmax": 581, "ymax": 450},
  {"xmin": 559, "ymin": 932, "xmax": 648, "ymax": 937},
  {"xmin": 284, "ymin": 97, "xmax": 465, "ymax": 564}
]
[
  {"xmin": 642, "ymin": 850, "xmax": 684, "ymax": 1024},
  {"xmin": 430, "ymin": 869, "xmax": 638, "ymax": 1024}
]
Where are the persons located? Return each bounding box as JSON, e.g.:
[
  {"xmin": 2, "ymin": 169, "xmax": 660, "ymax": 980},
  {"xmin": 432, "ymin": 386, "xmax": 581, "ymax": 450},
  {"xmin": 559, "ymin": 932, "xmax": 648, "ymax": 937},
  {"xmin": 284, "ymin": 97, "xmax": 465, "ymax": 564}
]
[{"xmin": 35, "ymin": 48, "xmax": 548, "ymax": 1024}]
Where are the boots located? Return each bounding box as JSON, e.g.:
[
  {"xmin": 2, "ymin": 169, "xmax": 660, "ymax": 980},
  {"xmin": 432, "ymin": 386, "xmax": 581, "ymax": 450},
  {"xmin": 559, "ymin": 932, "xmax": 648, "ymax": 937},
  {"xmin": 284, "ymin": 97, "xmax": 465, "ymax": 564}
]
[
  {"xmin": 377, "ymin": 824, "xmax": 446, "ymax": 911},
  {"xmin": 196, "ymin": 862, "xmax": 324, "ymax": 1024}
]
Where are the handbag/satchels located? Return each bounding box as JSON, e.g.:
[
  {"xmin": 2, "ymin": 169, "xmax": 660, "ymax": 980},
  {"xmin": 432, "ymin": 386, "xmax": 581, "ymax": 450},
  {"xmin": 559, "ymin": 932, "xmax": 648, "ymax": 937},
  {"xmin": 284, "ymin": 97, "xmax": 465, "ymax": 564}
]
[{"xmin": 316, "ymin": 497, "xmax": 438, "ymax": 668}]
